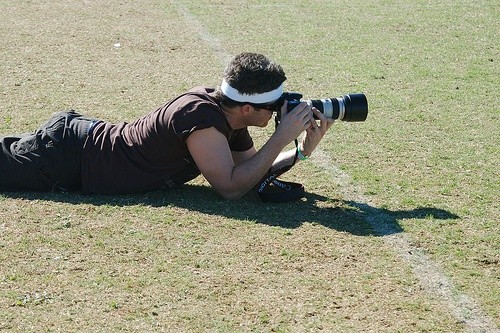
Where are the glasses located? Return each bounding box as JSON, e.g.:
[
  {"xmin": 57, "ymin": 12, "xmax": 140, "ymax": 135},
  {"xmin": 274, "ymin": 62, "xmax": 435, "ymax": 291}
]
[{"xmin": 256, "ymin": 102, "xmax": 275, "ymax": 111}]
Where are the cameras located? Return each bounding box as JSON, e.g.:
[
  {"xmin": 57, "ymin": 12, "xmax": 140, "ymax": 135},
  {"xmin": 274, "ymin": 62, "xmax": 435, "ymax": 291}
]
[{"xmin": 272, "ymin": 91, "xmax": 369, "ymax": 126}]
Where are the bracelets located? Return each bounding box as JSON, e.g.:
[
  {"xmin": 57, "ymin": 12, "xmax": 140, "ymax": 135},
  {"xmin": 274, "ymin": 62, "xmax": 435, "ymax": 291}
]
[{"xmin": 296, "ymin": 144, "xmax": 309, "ymax": 161}]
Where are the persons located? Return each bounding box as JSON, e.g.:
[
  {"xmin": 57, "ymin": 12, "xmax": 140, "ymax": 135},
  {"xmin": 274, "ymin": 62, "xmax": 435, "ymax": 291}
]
[{"xmin": 0, "ymin": 50, "xmax": 333, "ymax": 203}]
